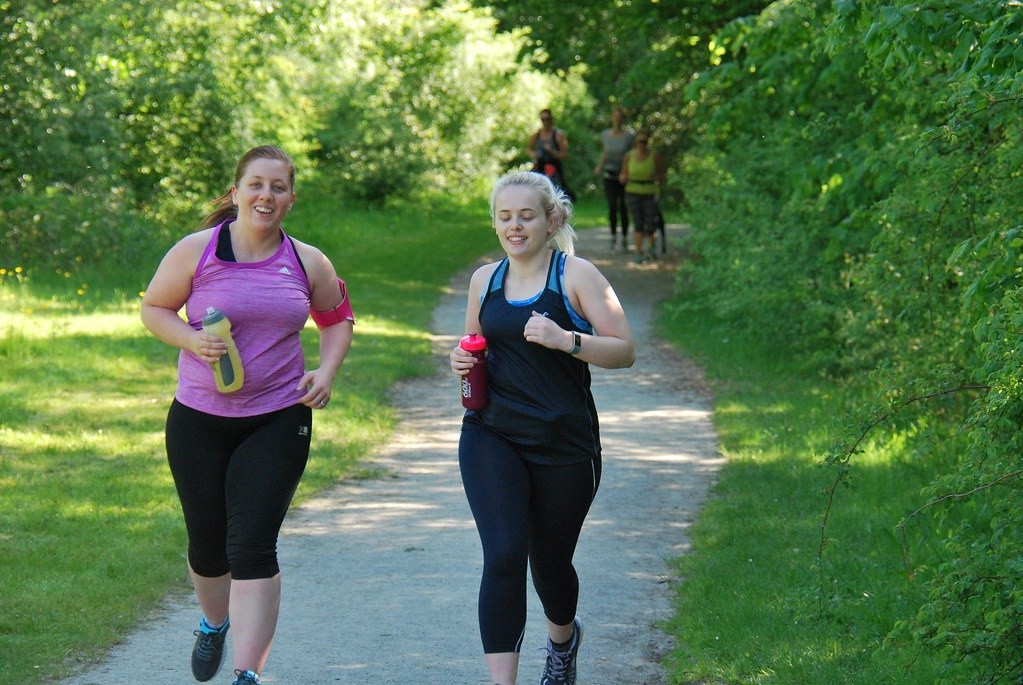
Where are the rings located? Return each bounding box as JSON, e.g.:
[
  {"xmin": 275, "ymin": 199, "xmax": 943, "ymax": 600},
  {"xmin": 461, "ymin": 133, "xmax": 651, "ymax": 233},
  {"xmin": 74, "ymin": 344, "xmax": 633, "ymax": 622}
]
[{"xmin": 321, "ymin": 400, "xmax": 324, "ymax": 405}]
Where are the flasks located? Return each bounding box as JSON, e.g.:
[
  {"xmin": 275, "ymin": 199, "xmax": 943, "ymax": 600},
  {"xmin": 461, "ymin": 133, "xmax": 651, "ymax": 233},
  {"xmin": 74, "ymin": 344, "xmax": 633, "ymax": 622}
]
[
  {"xmin": 459, "ymin": 332, "xmax": 488, "ymax": 410},
  {"xmin": 202, "ymin": 306, "xmax": 244, "ymax": 394}
]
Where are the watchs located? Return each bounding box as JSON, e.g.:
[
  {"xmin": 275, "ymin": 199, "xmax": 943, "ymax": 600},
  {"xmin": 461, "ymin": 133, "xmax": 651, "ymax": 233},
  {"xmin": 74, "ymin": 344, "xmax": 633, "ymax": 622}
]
[{"xmin": 568, "ymin": 331, "xmax": 581, "ymax": 354}]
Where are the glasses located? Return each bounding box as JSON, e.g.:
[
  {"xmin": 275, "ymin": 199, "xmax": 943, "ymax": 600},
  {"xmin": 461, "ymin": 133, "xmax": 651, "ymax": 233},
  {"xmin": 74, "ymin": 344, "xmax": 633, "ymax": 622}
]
[{"xmin": 637, "ymin": 140, "xmax": 648, "ymax": 144}]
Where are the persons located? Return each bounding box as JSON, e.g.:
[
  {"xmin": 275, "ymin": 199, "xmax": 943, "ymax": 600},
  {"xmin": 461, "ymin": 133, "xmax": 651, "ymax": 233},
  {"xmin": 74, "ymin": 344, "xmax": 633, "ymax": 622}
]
[
  {"xmin": 140, "ymin": 144, "xmax": 354, "ymax": 685},
  {"xmin": 450, "ymin": 171, "xmax": 637, "ymax": 685},
  {"xmin": 619, "ymin": 133, "xmax": 666, "ymax": 264},
  {"xmin": 527, "ymin": 108, "xmax": 576, "ymax": 205},
  {"xmin": 594, "ymin": 107, "xmax": 635, "ymax": 250}
]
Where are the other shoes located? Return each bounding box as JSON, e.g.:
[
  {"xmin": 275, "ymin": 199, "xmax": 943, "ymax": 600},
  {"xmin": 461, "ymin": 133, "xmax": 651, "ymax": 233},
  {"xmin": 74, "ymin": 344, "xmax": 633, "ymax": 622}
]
[
  {"xmin": 635, "ymin": 254, "xmax": 643, "ymax": 263},
  {"xmin": 649, "ymin": 246, "xmax": 656, "ymax": 255},
  {"xmin": 621, "ymin": 240, "xmax": 627, "ymax": 253},
  {"xmin": 610, "ymin": 240, "xmax": 615, "ymax": 249}
]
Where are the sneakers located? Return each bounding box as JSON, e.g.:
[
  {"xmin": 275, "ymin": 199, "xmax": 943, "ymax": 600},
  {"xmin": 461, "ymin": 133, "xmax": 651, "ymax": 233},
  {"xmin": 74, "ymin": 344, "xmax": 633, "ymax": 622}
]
[
  {"xmin": 190, "ymin": 615, "xmax": 231, "ymax": 681},
  {"xmin": 232, "ymin": 669, "xmax": 261, "ymax": 685},
  {"xmin": 537, "ymin": 616, "xmax": 585, "ymax": 685}
]
